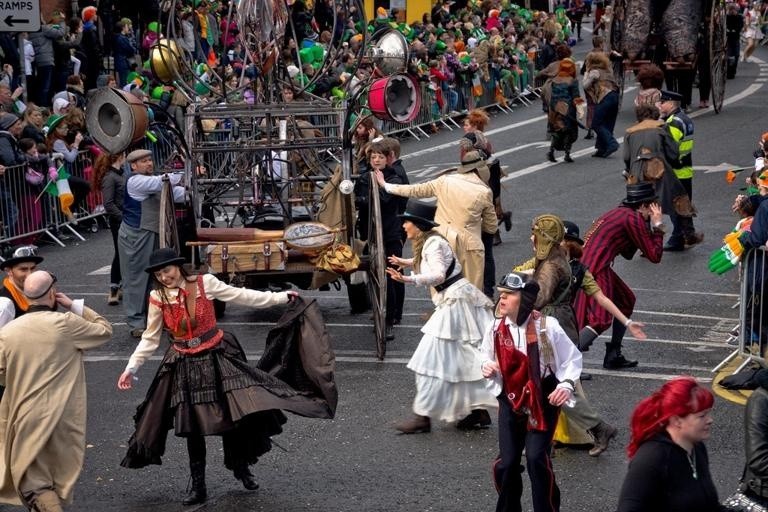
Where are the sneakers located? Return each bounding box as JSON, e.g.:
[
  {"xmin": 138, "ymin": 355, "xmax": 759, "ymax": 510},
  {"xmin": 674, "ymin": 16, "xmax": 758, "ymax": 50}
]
[
  {"xmin": 639, "ymin": 232, "xmax": 711, "ymax": 259},
  {"xmin": 586, "ymin": 417, "xmax": 617, "ymax": 457},
  {"xmin": 492, "ymin": 212, "xmax": 518, "ymax": 246},
  {"xmin": 106, "ymin": 279, "xmax": 127, "ymax": 307},
  {"xmin": 397, "ymin": 410, "xmax": 431, "ymax": 436},
  {"xmin": 682, "ymin": 97, "xmax": 716, "ymax": 118},
  {"xmin": 370, "ymin": 314, "xmax": 405, "ymax": 342},
  {"xmin": 539, "ymin": 142, "xmax": 620, "ymax": 165},
  {"xmin": 128, "ymin": 326, "xmax": 146, "ymax": 339},
  {"xmin": 460, "ymin": 408, "xmax": 494, "ymax": 432}
]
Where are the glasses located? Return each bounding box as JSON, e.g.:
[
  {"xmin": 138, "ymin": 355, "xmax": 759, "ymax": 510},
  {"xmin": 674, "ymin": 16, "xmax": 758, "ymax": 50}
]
[{"xmin": 499, "ymin": 274, "xmax": 526, "ymax": 290}]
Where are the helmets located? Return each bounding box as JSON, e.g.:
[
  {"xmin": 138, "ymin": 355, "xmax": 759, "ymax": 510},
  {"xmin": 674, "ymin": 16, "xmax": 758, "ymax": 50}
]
[{"xmin": 397, "ymin": 197, "xmax": 440, "ymax": 232}]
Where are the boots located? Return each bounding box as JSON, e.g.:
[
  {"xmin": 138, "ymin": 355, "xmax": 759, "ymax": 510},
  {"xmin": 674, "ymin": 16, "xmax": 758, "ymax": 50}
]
[
  {"xmin": 600, "ymin": 339, "xmax": 639, "ymax": 368},
  {"xmin": 579, "ymin": 325, "xmax": 597, "ymax": 382},
  {"xmin": 175, "ymin": 458, "xmax": 208, "ymax": 505},
  {"xmin": 232, "ymin": 458, "xmax": 262, "ymax": 493}
]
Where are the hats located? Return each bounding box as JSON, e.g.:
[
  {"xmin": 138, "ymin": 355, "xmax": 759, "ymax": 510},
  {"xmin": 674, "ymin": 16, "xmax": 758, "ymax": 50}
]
[
  {"xmin": 127, "ymin": 147, "xmax": 153, "ymax": 161},
  {"xmin": 562, "ymin": 220, "xmax": 586, "ymax": 247},
  {"xmin": 299, "ymin": 6, "xmax": 570, "ymax": 61},
  {"xmin": 146, "ymin": 246, "xmax": 185, "ymax": 275},
  {"xmin": 126, "ymin": 56, "xmax": 299, "ymax": 109},
  {"xmin": 458, "ymin": 151, "xmax": 492, "ymax": 184},
  {"xmin": 558, "ymin": 58, "xmax": 577, "ymax": 77},
  {"xmin": 494, "ymin": 271, "xmax": 540, "ymax": 328},
  {"xmin": 82, "ymin": 4, "xmax": 162, "ymax": 37},
  {"xmin": 47, "ymin": 112, "xmax": 67, "ymax": 136},
  {"xmin": 532, "ymin": 213, "xmax": 565, "ymax": 260},
  {"xmin": 0, "ymin": 245, "xmax": 46, "ymax": 271},
  {"xmin": 655, "ymin": 88, "xmax": 683, "ymax": 102},
  {"xmin": 1, "ymin": 110, "xmax": 20, "ymax": 129},
  {"xmin": 20, "ymin": 266, "xmax": 56, "ymax": 298},
  {"xmin": 620, "ymin": 181, "xmax": 660, "ymax": 205}
]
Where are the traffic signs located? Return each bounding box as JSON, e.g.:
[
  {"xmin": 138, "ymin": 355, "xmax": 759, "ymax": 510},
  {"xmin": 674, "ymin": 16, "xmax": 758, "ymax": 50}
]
[{"xmin": 0, "ymin": 0, "xmax": 41, "ymax": 32}]
